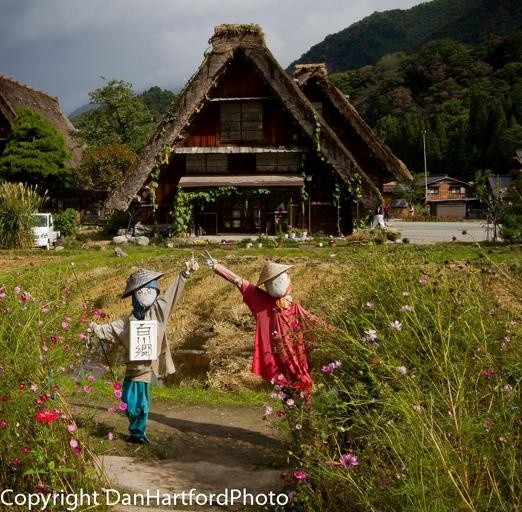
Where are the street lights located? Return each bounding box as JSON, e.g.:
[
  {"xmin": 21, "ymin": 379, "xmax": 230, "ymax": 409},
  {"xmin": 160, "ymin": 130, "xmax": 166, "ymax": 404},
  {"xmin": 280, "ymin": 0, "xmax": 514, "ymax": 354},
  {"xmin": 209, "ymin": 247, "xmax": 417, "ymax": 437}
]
[{"xmin": 421, "ymin": 129, "xmax": 428, "ymax": 208}]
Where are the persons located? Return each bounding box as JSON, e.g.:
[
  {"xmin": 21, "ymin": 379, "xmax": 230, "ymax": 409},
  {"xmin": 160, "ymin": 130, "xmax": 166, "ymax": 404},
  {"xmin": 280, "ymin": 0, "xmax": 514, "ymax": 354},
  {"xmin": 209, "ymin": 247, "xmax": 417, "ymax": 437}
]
[
  {"xmin": 204, "ymin": 249, "xmax": 341, "ymax": 441},
  {"xmin": 371, "ymin": 200, "xmax": 386, "ymax": 229},
  {"xmin": 90, "ymin": 252, "xmax": 204, "ymax": 443},
  {"xmin": 384, "ymin": 204, "xmax": 391, "ymax": 221},
  {"xmin": 408, "ymin": 204, "xmax": 415, "ymax": 216}
]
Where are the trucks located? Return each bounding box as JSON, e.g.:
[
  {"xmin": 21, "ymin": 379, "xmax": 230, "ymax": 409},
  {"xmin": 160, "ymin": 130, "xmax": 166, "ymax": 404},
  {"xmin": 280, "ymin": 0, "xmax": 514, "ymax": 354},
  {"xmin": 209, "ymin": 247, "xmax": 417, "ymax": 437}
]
[{"xmin": 29, "ymin": 212, "xmax": 61, "ymax": 250}]
